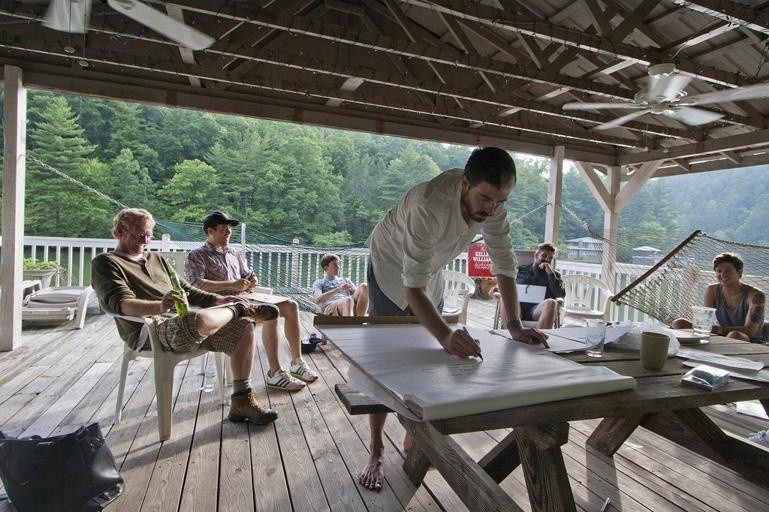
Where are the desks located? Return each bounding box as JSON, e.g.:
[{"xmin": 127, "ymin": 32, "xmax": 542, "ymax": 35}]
[{"xmin": 312, "ymin": 316, "xmax": 768, "ymax": 512}]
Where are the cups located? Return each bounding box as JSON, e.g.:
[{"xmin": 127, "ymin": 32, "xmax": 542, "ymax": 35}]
[
  {"xmin": 690, "ymin": 305, "xmax": 717, "ymax": 345},
  {"xmin": 585, "ymin": 320, "xmax": 606, "ymax": 358},
  {"xmin": 640, "ymin": 331, "xmax": 670, "ymax": 370}
]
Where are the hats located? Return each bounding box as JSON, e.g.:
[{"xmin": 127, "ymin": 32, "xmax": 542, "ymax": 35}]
[{"xmin": 202, "ymin": 211, "xmax": 240, "ymax": 233}]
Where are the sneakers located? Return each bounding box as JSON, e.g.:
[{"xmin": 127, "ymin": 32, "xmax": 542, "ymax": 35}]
[
  {"xmin": 747, "ymin": 430, "xmax": 769, "ymax": 449},
  {"xmin": 228, "ymin": 388, "xmax": 279, "ymax": 425},
  {"xmin": 290, "ymin": 361, "xmax": 319, "ymax": 383},
  {"xmin": 266, "ymin": 368, "xmax": 307, "ymax": 391}
]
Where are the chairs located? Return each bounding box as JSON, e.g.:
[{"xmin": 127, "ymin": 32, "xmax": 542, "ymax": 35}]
[
  {"xmin": 556, "ymin": 276, "xmax": 616, "ymax": 328},
  {"xmin": 439, "ymin": 268, "xmax": 477, "ymax": 327},
  {"xmin": 493, "ymin": 291, "xmax": 563, "ymax": 333}
]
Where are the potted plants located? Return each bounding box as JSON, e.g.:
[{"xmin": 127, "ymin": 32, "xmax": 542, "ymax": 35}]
[{"xmin": 23, "ymin": 257, "xmax": 61, "ymax": 288}]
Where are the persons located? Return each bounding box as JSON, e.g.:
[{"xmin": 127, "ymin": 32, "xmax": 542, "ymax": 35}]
[
  {"xmin": 310, "ymin": 251, "xmax": 368, "ymax": 316},
  {"xmin": 485, "ymin": 240, "xmax": 566, "ymax": 327},
  {"xmin": 180, "ymin": 210, "xmax": 319, "ymax": 392},
  {"xmin": 90, "ymin": 207, "xmax": 282, "ymax": 426},
  {"xmin": 669, "ymin": 252, "xmax": 766, "ymax": 342},
  {"xmin": 746, "ymin": 319, "xmax": 768, "ymax": 450},
  {"xmin": 358, "ymin": 145, "xmax": 549, "ymax": 491}
]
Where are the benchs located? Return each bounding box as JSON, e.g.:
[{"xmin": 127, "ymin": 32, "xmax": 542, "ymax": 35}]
[
  {"xmin": 96, "ymin": 251, "xmax": 237, "ymax": 442},
  {"xmin": 332, "ymin": 383, "xmax": 441, "ymax": 487}
]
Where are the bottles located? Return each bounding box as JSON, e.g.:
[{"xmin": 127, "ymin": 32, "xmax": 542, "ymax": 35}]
[
  {"xmin": 170, "ymin": 271, "xmax": 190, "ymax": 316},
  {"xmin": 203, "ymin": 353, "xmax": 215, "ymax": 392}
]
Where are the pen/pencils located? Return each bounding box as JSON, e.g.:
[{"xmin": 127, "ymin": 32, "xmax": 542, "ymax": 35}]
[{"xmin": 463, "ymin": 327, "xmax": 484, "ymax": 361}]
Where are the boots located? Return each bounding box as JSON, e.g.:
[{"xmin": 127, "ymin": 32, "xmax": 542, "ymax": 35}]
[{"xmin": 235, "ymin": 300, "xmax": 281, "ymax": 322}]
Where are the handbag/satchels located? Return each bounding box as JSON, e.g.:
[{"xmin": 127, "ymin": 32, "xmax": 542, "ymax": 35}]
[{"xmin": 0, "ymin": 422, "xmax": 127, "ymax": 512}]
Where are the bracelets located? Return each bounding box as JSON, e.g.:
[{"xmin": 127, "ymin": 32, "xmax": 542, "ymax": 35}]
[{"xmin": 503, "ymin": 319, "xmax": 523, "ymax": 332}]
